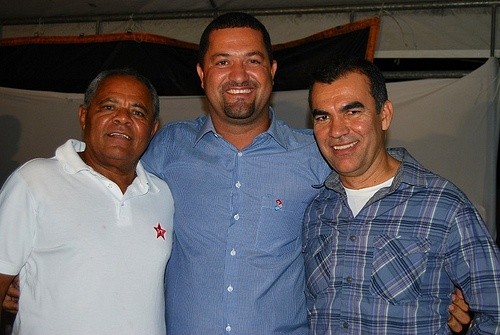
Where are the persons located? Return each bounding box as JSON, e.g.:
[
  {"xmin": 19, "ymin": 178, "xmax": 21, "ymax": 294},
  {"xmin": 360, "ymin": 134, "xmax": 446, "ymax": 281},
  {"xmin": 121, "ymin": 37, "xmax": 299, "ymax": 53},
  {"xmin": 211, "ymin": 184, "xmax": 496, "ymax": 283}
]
[
  {"xmin": 302, "ymin": 55, "xmax": 500, "ymax": 335},
  {"xmin": 1, "ymin": 12, "xmax": 470, "ymax": 335},
  {"xmin": 0, "ymin": 70, "xmax": 175, "ymax": 335}
]
